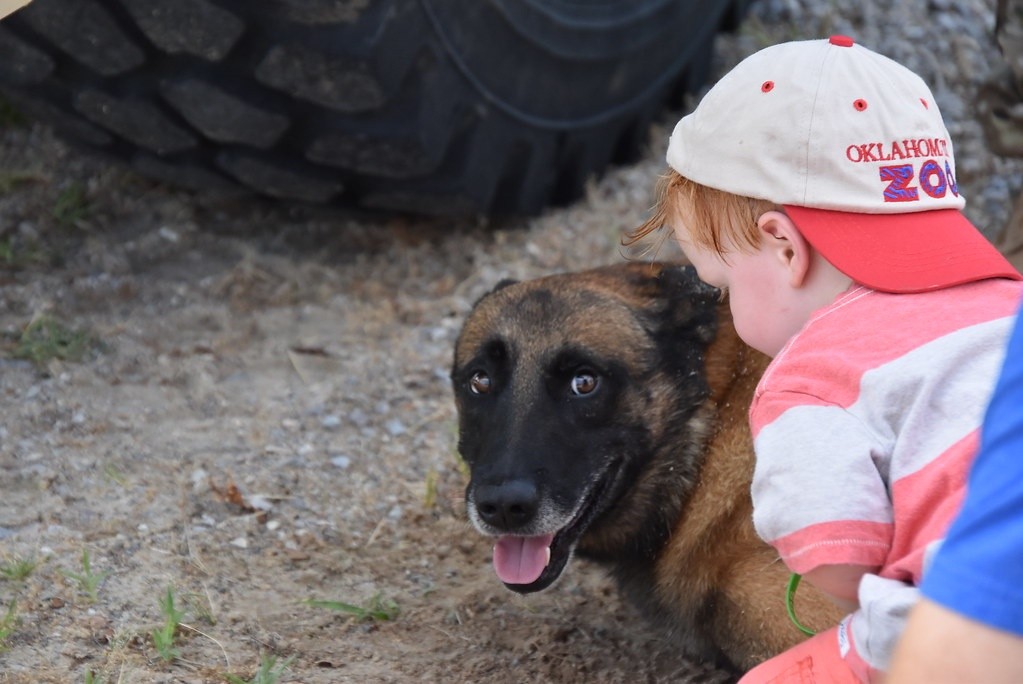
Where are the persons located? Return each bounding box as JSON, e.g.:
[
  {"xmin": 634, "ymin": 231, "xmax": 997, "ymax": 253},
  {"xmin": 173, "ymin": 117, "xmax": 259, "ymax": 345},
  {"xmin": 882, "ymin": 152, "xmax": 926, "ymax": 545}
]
[{"xmin": 656, "ymin": 37, "xmax": 1023, "ymax": 684}]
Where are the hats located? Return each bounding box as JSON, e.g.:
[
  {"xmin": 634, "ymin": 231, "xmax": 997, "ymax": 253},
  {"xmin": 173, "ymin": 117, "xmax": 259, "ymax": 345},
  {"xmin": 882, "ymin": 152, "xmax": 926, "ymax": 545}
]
[{"xmin": 666, "ymin": 36, "xmax": 1023, "ymax": 293}]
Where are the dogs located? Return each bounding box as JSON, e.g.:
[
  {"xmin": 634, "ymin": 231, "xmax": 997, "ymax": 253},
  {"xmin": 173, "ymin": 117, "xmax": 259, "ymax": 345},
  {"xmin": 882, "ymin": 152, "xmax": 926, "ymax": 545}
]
[{"xmin": 450, "ymin": 260, "xmax": 861, "ymax": 673}]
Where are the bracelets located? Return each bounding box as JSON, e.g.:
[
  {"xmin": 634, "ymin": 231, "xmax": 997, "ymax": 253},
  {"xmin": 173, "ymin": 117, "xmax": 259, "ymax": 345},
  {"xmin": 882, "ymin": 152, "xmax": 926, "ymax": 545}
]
[{"xmin": 786, "ymin": 572, "xmax": 816, "ymax": 637}]
